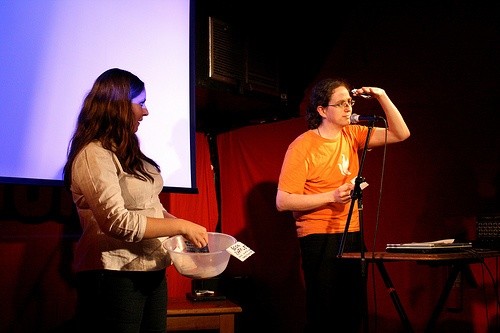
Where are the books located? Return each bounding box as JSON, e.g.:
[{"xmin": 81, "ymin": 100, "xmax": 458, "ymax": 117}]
[{"xmin": 387, "ymin": 238, "xmax": 472, "ymax": 247}]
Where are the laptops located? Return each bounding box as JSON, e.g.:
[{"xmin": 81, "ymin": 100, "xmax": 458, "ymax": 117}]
[{"xmin": 385, "ymin": 242, "xmax": 472, "ymax": 254}]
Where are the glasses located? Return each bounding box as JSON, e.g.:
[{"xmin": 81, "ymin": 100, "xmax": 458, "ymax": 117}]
[{"xmin": 327, "ymin": 100, "xmax": 355, "ymax": 108}]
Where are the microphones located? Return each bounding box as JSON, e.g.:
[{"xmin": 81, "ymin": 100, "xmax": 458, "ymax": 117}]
[{"xmin": 350, "ymin": 113, "xmax": 385, "ymax": 123}]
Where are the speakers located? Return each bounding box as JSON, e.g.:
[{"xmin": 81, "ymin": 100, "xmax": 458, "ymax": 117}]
[{"xmin": 191, "ymin": 276, "xmax": 266, "ymax": 333}]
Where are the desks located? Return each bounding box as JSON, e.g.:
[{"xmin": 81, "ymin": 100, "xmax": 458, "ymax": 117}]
[{"xmin": 339, "ymin": 251, "xmax": 500, "ymax": 333}]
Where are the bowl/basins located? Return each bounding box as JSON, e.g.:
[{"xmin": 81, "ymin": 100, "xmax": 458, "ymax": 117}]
[{"xmin": 162, "ymin": 227, "xmax": 238, "ymax": 280}]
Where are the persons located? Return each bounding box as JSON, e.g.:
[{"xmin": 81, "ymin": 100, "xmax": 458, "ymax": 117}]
[
  {"xmin": 276, "ymin": 80, "xmax": 410, "ymax": 333},
  {"xmin": 62, "ymin": 68, "xmax": 208, "ymax": 333}
]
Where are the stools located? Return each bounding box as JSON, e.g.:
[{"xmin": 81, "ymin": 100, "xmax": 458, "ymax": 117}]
[{"xmin": 166, "ymin": 300, "xmax": 243, "ymax": 333}]
